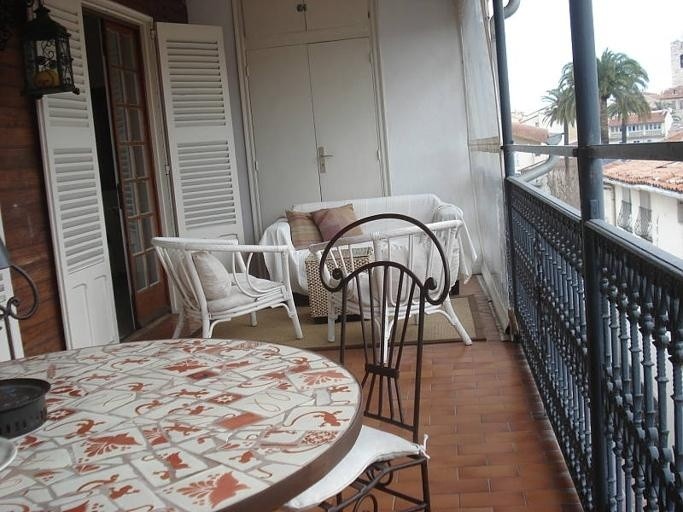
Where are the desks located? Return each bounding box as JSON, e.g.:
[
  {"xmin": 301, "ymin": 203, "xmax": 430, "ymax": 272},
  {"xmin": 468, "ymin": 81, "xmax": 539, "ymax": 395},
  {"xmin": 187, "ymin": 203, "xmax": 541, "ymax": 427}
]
[{"xmin": 0, "ymin": 336, "xmax": 363, "ymax": 512}]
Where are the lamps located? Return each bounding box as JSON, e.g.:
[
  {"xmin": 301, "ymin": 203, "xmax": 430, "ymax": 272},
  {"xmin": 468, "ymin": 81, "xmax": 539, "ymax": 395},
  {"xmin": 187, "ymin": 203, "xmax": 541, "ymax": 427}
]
[{"xmin": 0, "ymin": 0, "xmax": 81, "ymax": 99}]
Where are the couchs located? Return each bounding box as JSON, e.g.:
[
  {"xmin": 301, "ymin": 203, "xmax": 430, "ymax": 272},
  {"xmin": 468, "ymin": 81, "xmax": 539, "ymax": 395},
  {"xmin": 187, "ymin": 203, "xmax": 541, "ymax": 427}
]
[{"xmin": 263, "ymin": 191, "xmax": 464, "ymax": 302}]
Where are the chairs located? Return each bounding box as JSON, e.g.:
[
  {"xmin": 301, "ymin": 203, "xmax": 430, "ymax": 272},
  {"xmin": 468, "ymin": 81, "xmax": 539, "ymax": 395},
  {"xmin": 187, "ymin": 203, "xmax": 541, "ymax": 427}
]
[
  {"xmin": 309, "ymin": 220, "xmax": 471, "ymax": 364},
  {"xmin": 149, "ymin": 234, "xmax": 303, "ymax": 340},
  {"xmin": 318, "ymin": 213, "xmax": 451, "ymax": 512}
]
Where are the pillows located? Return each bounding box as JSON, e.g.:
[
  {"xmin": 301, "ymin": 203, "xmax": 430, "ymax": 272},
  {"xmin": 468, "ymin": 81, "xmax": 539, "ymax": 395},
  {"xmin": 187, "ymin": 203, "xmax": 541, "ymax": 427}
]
[
  {"xmin": 282, "ymin": 205, "xmax": 364, "ymax": 249},
  {"xmin": 177, "ymin": 252, "xmax": 231, "ymax": 301}
]
